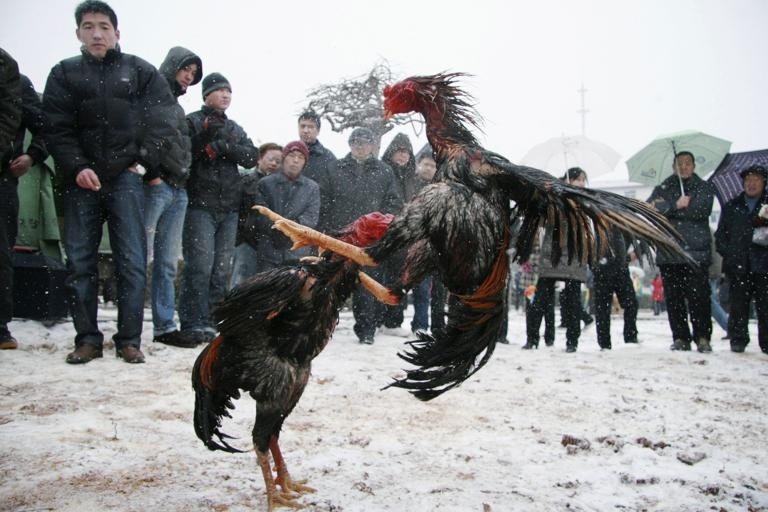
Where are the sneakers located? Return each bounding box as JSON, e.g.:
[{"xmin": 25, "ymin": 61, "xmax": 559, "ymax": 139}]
[
  {"xmin": 67, "ymin": 327, "xmax": 216, "ymax": 363},
  {"xmin": 355, "ymin": 322, "xmax": 511, "ymax": 344},
  {"xmin": 523, "ymin": 321, "xmax": 767, "ymax": 354}
]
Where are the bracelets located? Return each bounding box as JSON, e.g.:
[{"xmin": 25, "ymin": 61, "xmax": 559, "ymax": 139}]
[{"xmin": 132, "ymin": 163, "xmax": 148, "ymax": 177}]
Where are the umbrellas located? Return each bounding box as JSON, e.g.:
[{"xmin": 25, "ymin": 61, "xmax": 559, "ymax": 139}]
[
  {"xmin": 626, "ymin": 129, "xmax": 733, "ymax": 196},
  {"xmin": 517, "ymin": 132, "xmax": 626, "ymax": 185}
]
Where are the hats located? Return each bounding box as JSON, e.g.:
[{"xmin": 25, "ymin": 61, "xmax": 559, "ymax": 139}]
[
  {"xmin": 348, "ymin": 128, "xmax": 372, "ymax": 142},
  {"xmin": 202, "ymin": 72, "xmax": 231, "ymax": 101},
  {"xmin": 282, "ymin": 141, "xmax": 308, "ymax": 163}
]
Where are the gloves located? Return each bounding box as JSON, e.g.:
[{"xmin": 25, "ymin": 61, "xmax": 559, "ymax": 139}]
[
  {"xmin": 203, "ymin": 140, "xmax": 228, "ymax": 166},
  {"xmin": 202, "ymin": 110, "xmax": 224, "ymax": 138}
]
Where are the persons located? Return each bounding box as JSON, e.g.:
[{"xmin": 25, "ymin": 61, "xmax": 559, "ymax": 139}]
[
  {"xmin": 42, "ymin": 0, "xmax": 178, "ymax": 363},
  {"xmin": 496, "ymin": 167, "xmax": 638, "ymax": 354},
  {"xmin": 146, "ymin": 47, "xmax": 204, "ymax": 350},
  {"xmin": 1, "ymin": 44, "xmax": 48, "ymax": 348},
  {"xmin": 181, "ymin": 72, "xmax": 258, "ymax": 341},
  {"xmin": 229, "ymin": 111, "xmax": 447, "ymax": 344},
  {"xmin": 645, "ymin": 151, "xmax": 767, "ymax": 355}
]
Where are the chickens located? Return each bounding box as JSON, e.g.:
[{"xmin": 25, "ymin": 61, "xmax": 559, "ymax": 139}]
[
  {"xmin": 190, "ymin": 211, "xmax": 398, "ymax": 512},
  {"xmin": 248, "ymin": 69, "xmax": 701, "ymax": 403}
]
